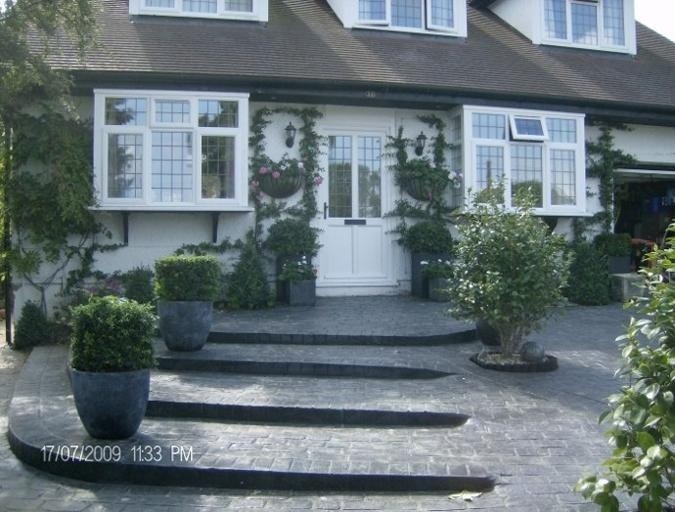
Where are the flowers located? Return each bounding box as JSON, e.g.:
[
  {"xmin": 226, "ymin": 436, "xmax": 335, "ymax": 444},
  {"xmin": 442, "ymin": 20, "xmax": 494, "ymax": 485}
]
[{"xmin": 248, "ymin": 152, "xmax": 325, "ymax": 186}]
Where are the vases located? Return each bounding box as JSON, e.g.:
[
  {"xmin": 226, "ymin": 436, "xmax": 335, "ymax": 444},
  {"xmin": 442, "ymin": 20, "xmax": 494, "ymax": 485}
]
[{"xmin": 256, "ymin": 176, "xmax": 302, "ymax": 198}]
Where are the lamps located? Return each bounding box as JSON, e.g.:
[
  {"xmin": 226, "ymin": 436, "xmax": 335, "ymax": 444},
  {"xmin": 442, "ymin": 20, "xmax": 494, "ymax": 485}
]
[
  {"xmin": 284, "ymin": 121, "xmax": 297, "ymax": 147},
  {"xmin": 415, "ymin": 130, "xmax": 428, "ymax": 157}
]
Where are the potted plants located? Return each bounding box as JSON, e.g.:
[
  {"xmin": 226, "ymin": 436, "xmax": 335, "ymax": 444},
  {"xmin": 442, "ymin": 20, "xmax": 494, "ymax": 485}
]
[
  {"xmin": 150, "ymin": 254, "xmax": 222, "ymax": 351},
  {"xmin": 64, "ymin": 294, "xmax": 159, "ymax": 440},
  {"xmin": 386, "ymin": 157, "xmax": 502, "ymax": 353},
  {"xmin": 255, "ymin": 216, "xmax": 325, "ymax": 306},
  {"xmin": 593, "ymin": 231, "xmax": 634, "ymax": 274}
]
